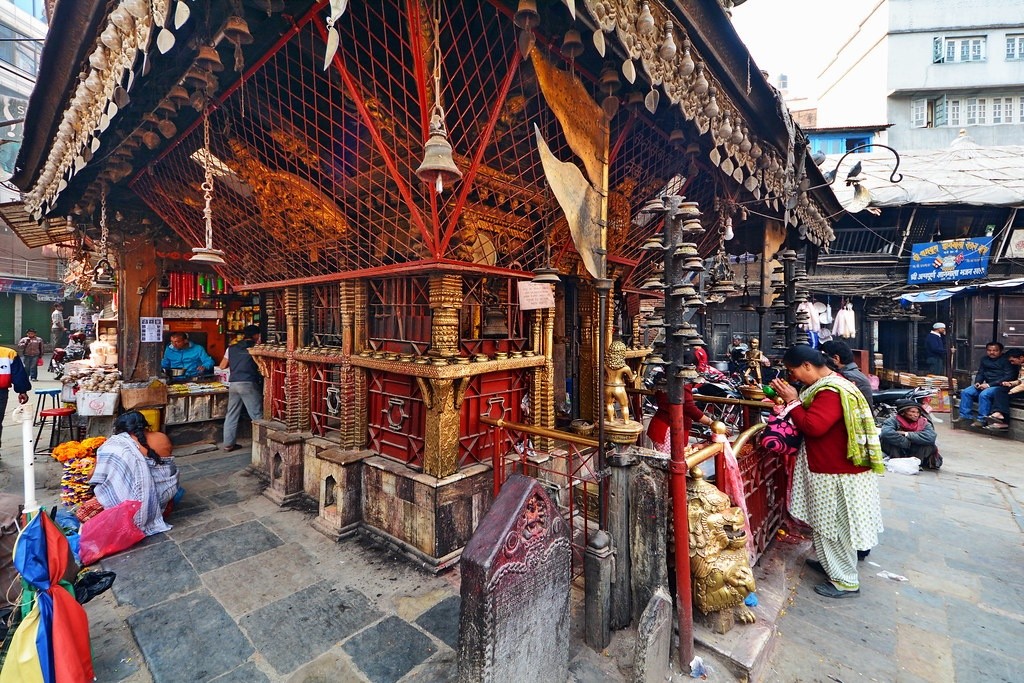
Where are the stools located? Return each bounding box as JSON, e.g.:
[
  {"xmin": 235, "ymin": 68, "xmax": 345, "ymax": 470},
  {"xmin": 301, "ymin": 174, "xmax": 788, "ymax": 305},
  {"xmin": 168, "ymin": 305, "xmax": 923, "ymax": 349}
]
[
  {"xmin": 34, "ymin": 409, "xmax": 76, "ymax": 460},
  {"xmin": 33, "ymin": 390, "xmax": 61, "ymax": 426}
]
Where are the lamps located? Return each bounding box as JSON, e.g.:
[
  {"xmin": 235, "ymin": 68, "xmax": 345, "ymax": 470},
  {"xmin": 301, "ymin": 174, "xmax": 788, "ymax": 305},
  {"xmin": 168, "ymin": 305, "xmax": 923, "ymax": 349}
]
[{"xmin": 66, "ymin": 216, "xmax": 75, "ymax": 231}]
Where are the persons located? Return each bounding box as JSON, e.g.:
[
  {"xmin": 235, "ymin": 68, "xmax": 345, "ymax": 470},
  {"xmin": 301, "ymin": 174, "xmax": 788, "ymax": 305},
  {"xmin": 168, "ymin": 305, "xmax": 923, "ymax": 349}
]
[
  {"xmin": 951, "ymin": 342, "xmax": 1024, "ymax": 432},
  {"xmin": 220, "ymin": 324, "xmax": 264, "ymax": 452},
  {"xmin": 18, "ymin": 328, "xmax": 44, "ymax": 381},
  {"xmin": 89, "ymin": 410, "xmax": 180, "ymax": 536},
  {"xmin": 51, "ymin": 302, "xmax": 70, "ymax": 351},
  {"xmin": 925, "ymin": 322, "xmax": 957, "ymax": 376},
  {"xmin": 771, "ymin": 341, "xmax": 887, "ymax": 596},
  {"xmin": 744, "ymin": 338, "xmax": 764, "ymax": 385},
  {"xmin": 160, "ymin": 331, "xmax": 215, "ymax": 380},
  {"xmin": 879, "ymin": 399, "xmax": 937, "ymax": 470},
  {"xmin": 604, "ymin": 341, "xmax": 638, "ymax": 424},
  {"xmin": 0, "ymin": 347, "xmax": 32, "ymax": 460},
  {"xmin": 647, "ymin": 335, "xmax": 747, "ymax": 459}
]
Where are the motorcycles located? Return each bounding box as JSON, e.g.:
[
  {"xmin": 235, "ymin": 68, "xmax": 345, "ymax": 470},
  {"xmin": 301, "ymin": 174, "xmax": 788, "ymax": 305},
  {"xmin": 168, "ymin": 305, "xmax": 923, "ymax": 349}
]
[{"xmin": 48, "ymin": 341, "xmax": 90, "ymax": 380}]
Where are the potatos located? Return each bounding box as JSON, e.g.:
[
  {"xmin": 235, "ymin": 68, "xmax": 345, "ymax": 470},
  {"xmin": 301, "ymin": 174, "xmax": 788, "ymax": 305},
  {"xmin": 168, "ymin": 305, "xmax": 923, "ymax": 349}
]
[{"xmin": 81, "ymin": 373, "xmax": 121, "ymax": 393}]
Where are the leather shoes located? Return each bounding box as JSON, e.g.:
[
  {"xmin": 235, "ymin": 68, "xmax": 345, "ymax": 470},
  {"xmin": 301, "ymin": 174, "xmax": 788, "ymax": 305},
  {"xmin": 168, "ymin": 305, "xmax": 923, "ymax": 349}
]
[
  {"xmin": 814, "ymin": 582, "xmax": 860, "ymax": 598},
  {"xmin": 806, "ymin": 558, "xmax": 825, "ymax": 574}
]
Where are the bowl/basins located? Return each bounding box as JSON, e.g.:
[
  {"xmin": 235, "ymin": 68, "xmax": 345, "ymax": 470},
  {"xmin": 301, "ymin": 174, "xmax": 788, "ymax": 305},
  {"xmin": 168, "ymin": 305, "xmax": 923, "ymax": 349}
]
[
  {"xmin": 711, "ymin": 360, "xmax": 728, "ymax": 371},
  {"xmin": 166, "ymin": 367, "xmax": 188, "ymax": 377}
]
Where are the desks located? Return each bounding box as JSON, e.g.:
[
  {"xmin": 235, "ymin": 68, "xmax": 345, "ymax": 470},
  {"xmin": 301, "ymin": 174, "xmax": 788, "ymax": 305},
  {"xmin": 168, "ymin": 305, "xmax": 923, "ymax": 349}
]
[{"xmin": 161, "ymin": 391, "xmax": 229, "ymax": 433}]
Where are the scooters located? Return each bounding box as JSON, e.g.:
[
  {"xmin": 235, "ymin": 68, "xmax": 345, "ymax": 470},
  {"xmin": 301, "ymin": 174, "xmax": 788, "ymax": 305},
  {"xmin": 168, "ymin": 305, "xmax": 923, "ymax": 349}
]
[
  {"xmin": 871, "ymin": 386, "xmax": 935, "ymax": 431},
  {"xmin": 643, "ymin": 366, "xmax": 744, "ymax": 434}
]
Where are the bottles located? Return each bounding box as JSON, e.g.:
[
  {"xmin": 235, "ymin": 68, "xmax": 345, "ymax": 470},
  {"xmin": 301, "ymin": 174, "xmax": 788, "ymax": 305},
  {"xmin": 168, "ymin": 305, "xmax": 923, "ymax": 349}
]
[
  {"xmin": 210, "ymin": 297, "xmax": 223, "ymax": 309},
  {"xmin": 219, "ymin": 321, "xmax": 222, "ymax": 334}
]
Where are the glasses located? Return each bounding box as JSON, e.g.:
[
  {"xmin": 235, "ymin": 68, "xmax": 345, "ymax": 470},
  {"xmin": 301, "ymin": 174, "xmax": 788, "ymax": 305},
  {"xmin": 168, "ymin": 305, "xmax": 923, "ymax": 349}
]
[{"xmin": 789, "ymin": 368, "xmax": 797, "ymax": 375}]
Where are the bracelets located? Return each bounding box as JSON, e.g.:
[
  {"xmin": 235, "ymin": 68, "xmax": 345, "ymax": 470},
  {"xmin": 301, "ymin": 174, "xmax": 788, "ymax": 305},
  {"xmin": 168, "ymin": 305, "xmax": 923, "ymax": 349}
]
[
  {"xmin": 787, "ymin": 399, "xmax": 798, "ymax": 404},
  {"xmin": 706, "ymin": 418, "xmax": 711, "ymax": 425}
]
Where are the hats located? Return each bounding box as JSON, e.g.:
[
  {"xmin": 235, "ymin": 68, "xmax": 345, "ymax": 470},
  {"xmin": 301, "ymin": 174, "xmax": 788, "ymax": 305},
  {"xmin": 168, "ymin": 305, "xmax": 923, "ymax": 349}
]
[{"xmin": 932, "ymin": 322, "xmax": 946, "ymax": 329}]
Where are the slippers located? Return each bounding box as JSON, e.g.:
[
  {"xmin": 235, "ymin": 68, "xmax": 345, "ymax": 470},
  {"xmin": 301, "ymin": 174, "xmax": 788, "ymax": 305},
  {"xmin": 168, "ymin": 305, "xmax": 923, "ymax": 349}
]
[
  {"xmin": 982, "ymin": 423, "xmax": 1008, "ymax": 432},
  {"xmin": 983, "ymin": 415, "xmax": 1005, "ymax": 423}
]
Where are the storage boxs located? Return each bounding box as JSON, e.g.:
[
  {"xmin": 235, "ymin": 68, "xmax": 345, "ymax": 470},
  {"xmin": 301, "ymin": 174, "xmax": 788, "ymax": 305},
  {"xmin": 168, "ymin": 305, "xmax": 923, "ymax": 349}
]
[{"xmin": 63, "ymin": 320, "xmax": 167, "ymax": 415}]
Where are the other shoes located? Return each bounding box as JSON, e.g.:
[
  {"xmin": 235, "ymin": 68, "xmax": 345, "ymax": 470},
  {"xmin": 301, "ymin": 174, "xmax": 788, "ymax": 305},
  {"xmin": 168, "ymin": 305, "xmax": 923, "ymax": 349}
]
[
  {"xmin": 950, "ymin": 416, "xmax": 965, "ymax": 424},
  {"xmin": 224, "ymin": 444, "xmax": 242, "ymax": 452},
  {"xmin": 970, "ymin": 420, "xmax": 984, "ymax": 427}
]
